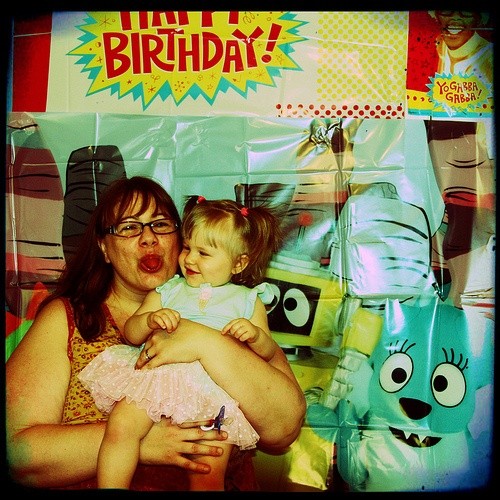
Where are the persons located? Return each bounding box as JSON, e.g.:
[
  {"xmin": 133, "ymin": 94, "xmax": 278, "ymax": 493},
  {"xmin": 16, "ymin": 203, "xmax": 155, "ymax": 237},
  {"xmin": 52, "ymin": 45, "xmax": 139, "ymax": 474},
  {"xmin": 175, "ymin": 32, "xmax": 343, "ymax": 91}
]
[
  {"xmin": 6, "ymin": 176, "xmax": 307, "ymax": 492},
  {"xmin": 77, "ymin": 195, "xmax": 290, "ymax": 491}
]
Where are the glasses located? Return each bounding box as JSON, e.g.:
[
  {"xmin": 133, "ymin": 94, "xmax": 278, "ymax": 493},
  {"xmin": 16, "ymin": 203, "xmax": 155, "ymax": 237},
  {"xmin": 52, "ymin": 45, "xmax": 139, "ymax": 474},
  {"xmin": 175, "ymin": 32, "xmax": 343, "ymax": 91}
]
[{"xmin": 100, "ymin": 218, "xmax": 184, "ymax": 237}]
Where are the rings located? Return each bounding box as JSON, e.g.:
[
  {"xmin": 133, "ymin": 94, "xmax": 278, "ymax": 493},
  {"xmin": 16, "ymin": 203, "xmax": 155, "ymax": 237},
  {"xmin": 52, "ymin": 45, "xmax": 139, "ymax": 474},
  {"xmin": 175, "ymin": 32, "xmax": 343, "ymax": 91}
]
[{"xmin": 146, "ymin": 349, "xmax": 151, "ymax": 360}]
